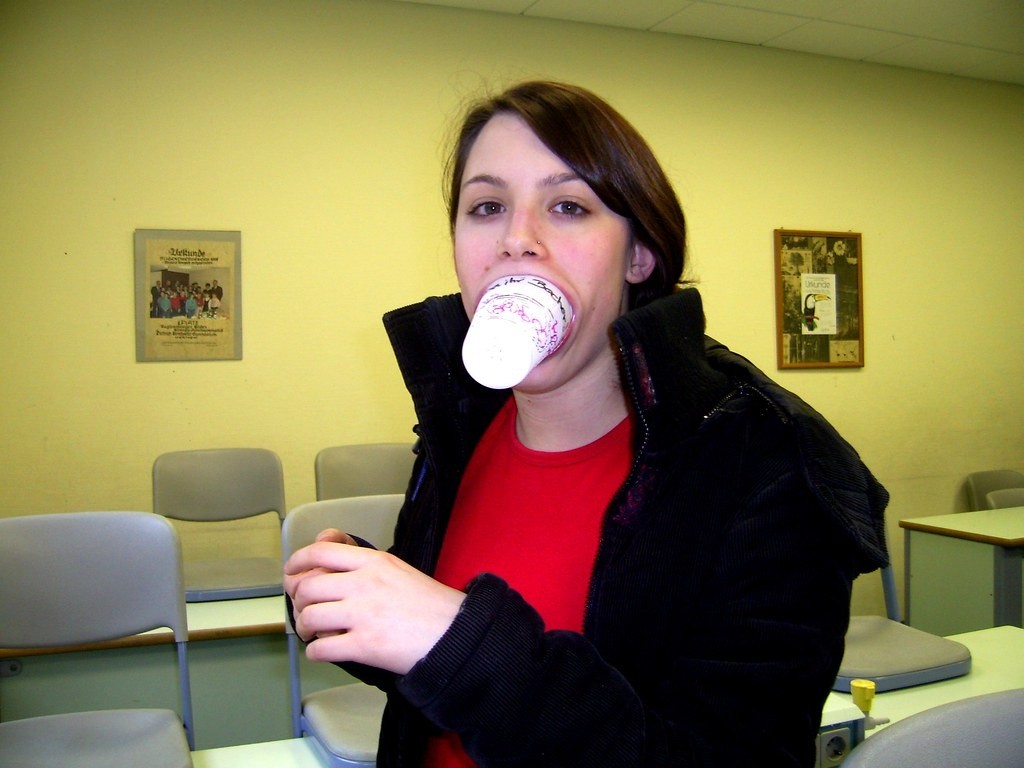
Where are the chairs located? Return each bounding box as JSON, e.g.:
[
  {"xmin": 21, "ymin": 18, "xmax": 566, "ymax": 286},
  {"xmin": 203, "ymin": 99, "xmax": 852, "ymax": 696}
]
[
  {"xmin": 839, "ymin": 686, "xmax": 1024, "ymax": 768},
  {"xmin": 151, "ymin": 446, "xmax": 291, "ymax": 600},
  {"xmin": 280, "ymin": 496, "xmax": 404, "ymax": 768},
  {"xmin": 831, "ymin": 563, "xmax": 971, "ymax": 696},
  {"xmin": 0, "ymin": 511, "xmax": 198, "ymax": 768},
  {"xmin": 967, "ymin": 469, "xmax": 1024, "ymax": 512},
  {"xmin": 314, "ymin": 442, "xmax": 418, "ymax": 500}
]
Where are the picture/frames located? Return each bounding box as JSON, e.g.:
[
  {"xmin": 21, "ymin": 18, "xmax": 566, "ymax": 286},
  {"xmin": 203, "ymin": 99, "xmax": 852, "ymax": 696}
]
[{"xmin": 774, "ymin": 229, "xmax": 865, "ymax": 371}]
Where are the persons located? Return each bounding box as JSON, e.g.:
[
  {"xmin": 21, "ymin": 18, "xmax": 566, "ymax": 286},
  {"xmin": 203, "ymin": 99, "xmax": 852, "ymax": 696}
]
[
  {"xmin": 278, "ymin": 83, "xmax": 889, "ymax": 768},
  {"xmin": 150, "ymin": 279, "xmax": 224, "ymax": 319}
]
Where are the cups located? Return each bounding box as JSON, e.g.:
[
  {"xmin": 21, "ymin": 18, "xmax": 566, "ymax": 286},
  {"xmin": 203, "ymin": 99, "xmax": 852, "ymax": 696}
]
[{"xmin": 462, "ymin": 276, "xmax": 572, "ymax": 390}]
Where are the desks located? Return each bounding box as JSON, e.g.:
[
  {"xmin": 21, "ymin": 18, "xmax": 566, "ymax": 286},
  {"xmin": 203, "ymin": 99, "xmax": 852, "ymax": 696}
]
[
  {"xmin": 898, "ymin": 504, "xmax": 1024, "ymax": 637},
  {"xmin": 0, "ymin": 594, "xmax": 359, "ymax": 749},
  {"xmin": 190, "ymin": 623, "xmax": 1024, "ymax": 768}
]
